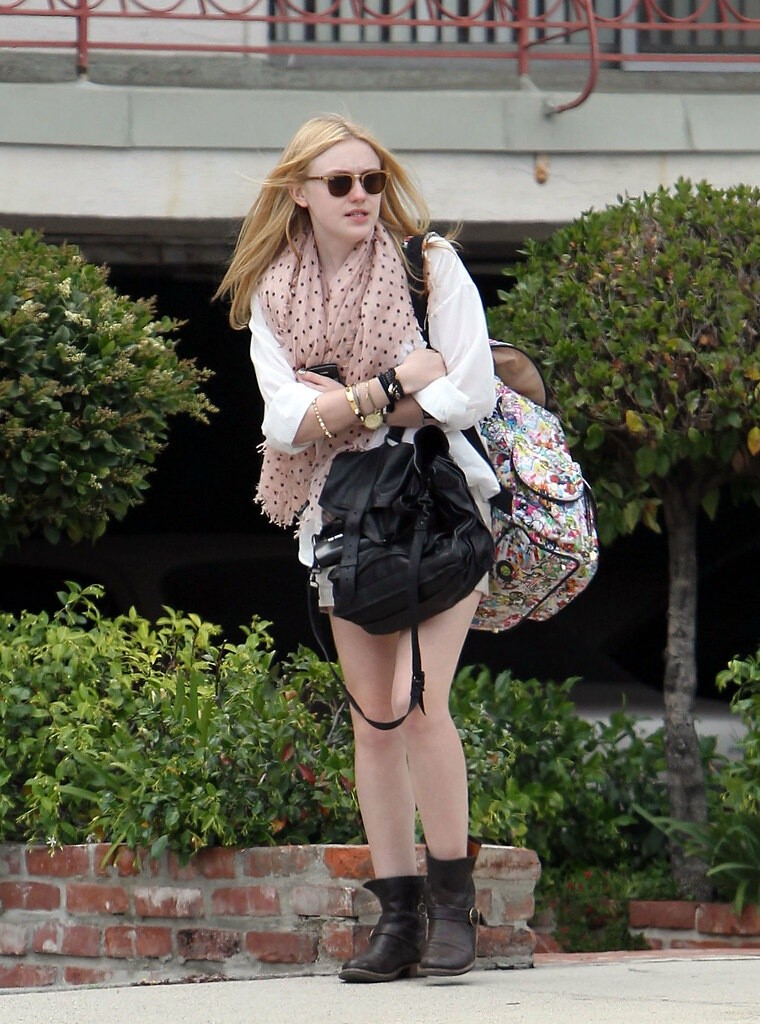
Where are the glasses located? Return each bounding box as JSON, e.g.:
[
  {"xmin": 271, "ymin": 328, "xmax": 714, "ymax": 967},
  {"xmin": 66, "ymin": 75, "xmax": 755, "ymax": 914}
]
[{"xmin": 306, "ymin": 170, "xmax": 392, "ymax": 197}]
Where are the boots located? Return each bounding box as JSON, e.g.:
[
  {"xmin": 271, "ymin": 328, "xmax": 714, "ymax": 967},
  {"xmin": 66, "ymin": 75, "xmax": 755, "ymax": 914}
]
[
  {"xmin": 339, "ymin": 875, "xmax": 428, "ymax": 982},
  {"xmin": 417, "ymin": 834, "xmax": 483, "ymax": 976}
]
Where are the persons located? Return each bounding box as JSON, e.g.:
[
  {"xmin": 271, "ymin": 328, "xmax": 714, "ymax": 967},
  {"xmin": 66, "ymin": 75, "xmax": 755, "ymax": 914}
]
[{"xmin": 213, "ymin": 114, "xmax": 501, "ymax": 981}]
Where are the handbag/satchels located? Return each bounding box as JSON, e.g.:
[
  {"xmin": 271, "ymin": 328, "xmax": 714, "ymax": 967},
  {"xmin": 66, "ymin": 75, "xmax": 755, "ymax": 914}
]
[{"xmin": 310, "ymin": 425, "xmax": 494, "ymax": 731}]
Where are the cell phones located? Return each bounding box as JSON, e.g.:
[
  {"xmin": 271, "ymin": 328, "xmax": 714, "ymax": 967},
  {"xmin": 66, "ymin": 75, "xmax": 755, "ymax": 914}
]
[{"xmin": 306, "ymin": 364, "xmax": 341, "ymax": 387}]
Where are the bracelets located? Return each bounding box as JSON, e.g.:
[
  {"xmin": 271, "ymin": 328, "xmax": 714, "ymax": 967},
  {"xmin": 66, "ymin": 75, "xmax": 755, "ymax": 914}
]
[
  {"xmin": 312, "ymin": 400, "xmax": 333, "ymax": 438},
  {"xmin": 345, "ymin": 381, "xmax": 383, "ymax": 430},
  {"xmin": 378, "ymin": 366, "xmax": 405, "ymax": 409}
]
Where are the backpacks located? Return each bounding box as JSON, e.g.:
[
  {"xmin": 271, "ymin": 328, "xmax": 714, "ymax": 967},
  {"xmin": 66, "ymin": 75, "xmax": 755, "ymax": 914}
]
[{"xmin": 408, "ymin": 233, "xmax": 599, "ymax": 634}]
